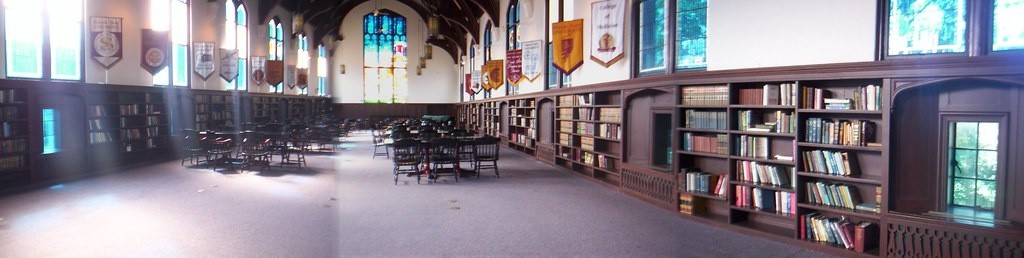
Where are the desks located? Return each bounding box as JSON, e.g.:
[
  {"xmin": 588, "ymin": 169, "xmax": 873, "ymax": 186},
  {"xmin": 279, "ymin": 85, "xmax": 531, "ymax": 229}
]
[
  {"xmin": 415, "ymin": 136, "xmax": 485, "ymax": 179},
  {"xmin": 199, "ymin": 131, "xmax": 289, "ymax": 167}
]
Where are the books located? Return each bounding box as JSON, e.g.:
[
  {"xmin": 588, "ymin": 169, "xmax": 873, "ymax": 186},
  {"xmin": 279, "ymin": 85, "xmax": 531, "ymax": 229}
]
[
  {"xmin": 253, "ymin": 97, "xmax": 275, "ymax": 116},
  {"xmin": 89, "ymin": 106, "xmax": 160, "ymax": 151},
  {"xmin": 678, "ymin": 83, "xmax": 882, "ymax": 254},
  {"xmin": 0, "ymin": 89, "xmax": 27, "ymax": 169},
  {"xmin": 510, "ymin": 100, "xmax": 535, "ymax": 146},
  {"xmin": 559, "ymin": 94, "xmax": 621, "ymax": 170},
  {"xmin": 196, "ymin": 96, "xmax": 232, "ymax": 129},
  {"xmin": 469, "ymin": 104, "xmax": 479, "ymax": 125},
  {"xmin": 485, "ymin": 102, "xmax": 499, "ymax": 137}
]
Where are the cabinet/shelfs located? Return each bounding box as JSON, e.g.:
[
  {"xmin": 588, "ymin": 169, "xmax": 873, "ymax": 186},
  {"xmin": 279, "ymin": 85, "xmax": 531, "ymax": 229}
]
[
  {"xmin": 456, "ymin": 60, "xmax": 887, "ymax": 258},
  {"xmin": 0, "ymin": 78, "xmax": 332, "ymax": 193}
]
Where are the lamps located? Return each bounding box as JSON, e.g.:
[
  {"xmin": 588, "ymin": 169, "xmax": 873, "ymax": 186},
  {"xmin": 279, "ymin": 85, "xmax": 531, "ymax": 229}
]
[{"xmin": 289, "ymin": 0, "xmax": 439, "ymax": 75}]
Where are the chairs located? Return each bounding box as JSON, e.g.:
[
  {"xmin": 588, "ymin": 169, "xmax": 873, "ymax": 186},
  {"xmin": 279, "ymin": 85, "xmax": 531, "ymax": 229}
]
[{"xmin": 177, "ymin": 113, "xmax": 502, "ymax": 184}]
